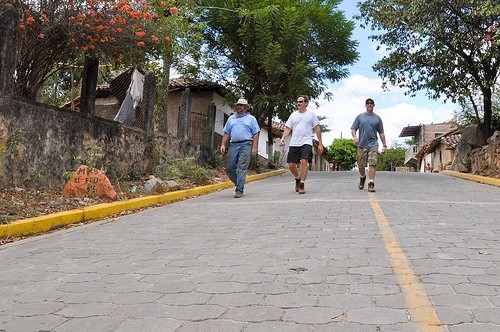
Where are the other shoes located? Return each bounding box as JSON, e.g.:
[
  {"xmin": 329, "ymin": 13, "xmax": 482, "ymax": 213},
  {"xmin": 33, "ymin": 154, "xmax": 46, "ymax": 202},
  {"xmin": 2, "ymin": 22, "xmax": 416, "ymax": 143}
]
[{"xmin": 234, "ymin": 191, "xmax": 244, "ymax": 197}]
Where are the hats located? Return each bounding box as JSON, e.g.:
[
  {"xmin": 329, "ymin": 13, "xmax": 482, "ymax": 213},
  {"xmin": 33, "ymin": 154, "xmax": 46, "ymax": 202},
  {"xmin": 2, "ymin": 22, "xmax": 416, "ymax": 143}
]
[
  {"xmin": 234, "ymin": 99, "xmax": 250, "ymax": 110},
  {"xmin": 366, "ymin": 99, "xmax": 374, "ymax": 102}
]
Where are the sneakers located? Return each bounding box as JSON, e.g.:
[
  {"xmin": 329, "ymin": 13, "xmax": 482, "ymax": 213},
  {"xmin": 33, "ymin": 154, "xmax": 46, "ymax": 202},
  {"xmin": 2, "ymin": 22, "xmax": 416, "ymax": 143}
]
[
  {"xmin": 368, "ymin": 182, "xmax": 375, "ymax": 191},
  {"xmin": 295, "ymin": 177, "xmax": 301, "ymax": 192},
  {"xmin": 359, "ymin": 175, "xmax": 366, "ymax": 189},
  {"xmin": 299, "ymin": 182, "xmax": 305, "ymax": 193}
]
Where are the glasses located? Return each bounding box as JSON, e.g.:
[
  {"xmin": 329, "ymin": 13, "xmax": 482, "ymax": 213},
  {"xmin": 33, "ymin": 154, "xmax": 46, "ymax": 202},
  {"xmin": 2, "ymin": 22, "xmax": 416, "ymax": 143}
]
[
  {"xmin": 235, "ymin": 105, "xmax": 245, "ymax": 107},
  {"xmin": 297, "ymin": 101, "xmax": 304, "ymax": 103},
  {"xmin": 366, "ymin": 103, "xmax": 373, "ymax": 104}
]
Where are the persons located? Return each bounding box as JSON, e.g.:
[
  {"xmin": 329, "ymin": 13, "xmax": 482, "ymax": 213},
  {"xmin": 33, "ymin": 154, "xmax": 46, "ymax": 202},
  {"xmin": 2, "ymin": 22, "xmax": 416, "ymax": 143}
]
[
  {"xmin": 279, "ymin": 95, "xmax": 323, "ymax": 194},
  {"xmin": 220, "ymin": 99, "xmax": 261, "ymax": 198},
  {"xmin": 350, "ymin": 98, "xmax": 387, "ymax": 192}
]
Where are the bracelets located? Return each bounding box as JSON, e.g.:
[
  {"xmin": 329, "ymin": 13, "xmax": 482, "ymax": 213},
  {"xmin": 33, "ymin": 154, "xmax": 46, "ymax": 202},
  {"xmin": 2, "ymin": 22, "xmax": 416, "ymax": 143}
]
[{"xmin": 383, "ymin": 146, "xmax": 387, "ymax": 147}]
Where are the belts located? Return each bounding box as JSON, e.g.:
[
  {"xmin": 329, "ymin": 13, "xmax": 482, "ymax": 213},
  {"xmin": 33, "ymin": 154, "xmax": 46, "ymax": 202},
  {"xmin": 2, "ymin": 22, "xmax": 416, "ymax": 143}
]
[{"xmin": 230, "ymin": 140, "xmax": 252, "ymax": 143}]
